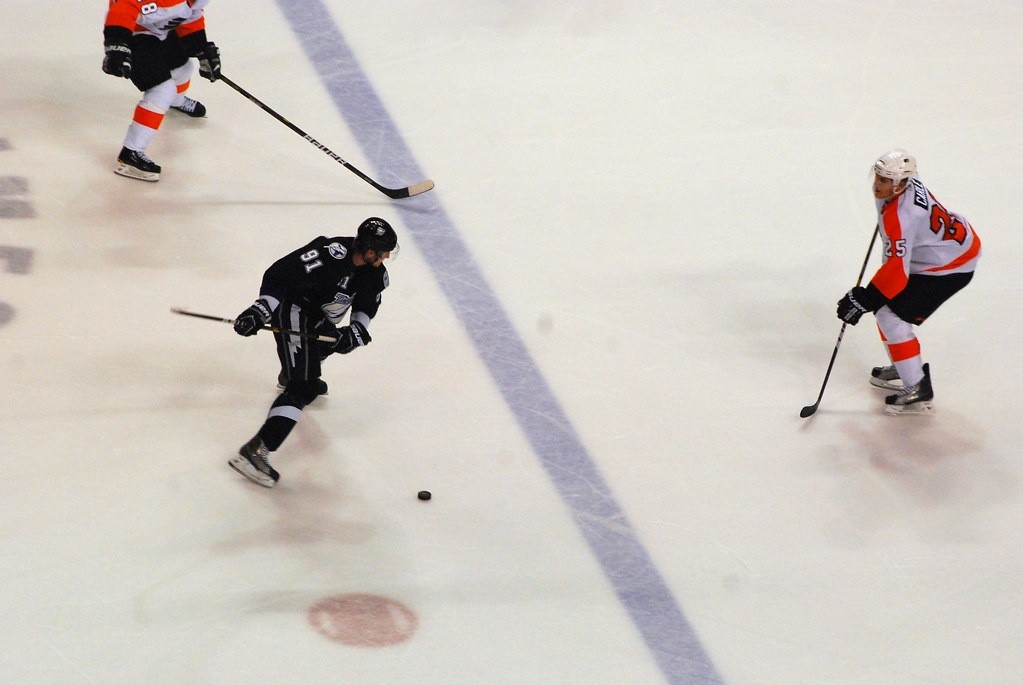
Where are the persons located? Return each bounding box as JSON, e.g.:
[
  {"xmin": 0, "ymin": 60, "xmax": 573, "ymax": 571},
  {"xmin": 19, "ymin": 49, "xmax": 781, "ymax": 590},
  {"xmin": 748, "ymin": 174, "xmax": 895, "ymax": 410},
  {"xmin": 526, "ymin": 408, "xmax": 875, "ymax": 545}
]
[
  {"xmin": 836, "ymin": 149, "xmax": 982, "ymax": 415},
  {"xmin": 228, "ymin": 216, "xmax": 400, "ymax": 488},
  {"xmin": 100, "ymin": 0, "xmax": 221, "ymax": 183}
]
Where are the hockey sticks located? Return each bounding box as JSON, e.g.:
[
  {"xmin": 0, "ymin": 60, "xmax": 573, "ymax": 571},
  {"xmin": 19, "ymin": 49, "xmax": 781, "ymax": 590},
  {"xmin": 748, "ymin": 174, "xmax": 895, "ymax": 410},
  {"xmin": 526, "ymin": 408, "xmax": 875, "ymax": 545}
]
[
  {"xmin": 216, "ymin": 72, "xmax": 435, "ymax": 199},
  {"xmin": 170, "ymin": 307, "xmax": 338, "ymax": 344},
  {"xmin": 799, "ymin": 225, "xmax": 879, "ymax": 418}
]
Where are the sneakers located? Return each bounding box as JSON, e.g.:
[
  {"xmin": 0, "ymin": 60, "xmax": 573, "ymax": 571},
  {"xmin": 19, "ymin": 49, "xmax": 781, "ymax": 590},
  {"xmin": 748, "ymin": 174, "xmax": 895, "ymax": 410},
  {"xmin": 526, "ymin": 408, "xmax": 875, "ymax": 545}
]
[
  {"xmin": 114, "ymin": 147, "xmax": 161, "ymax": 181},
  {"xmin": 228, "ymin": 434, "xmax": 280, "ymax": 488},
  {"xmin": 169, "ymin": 96, "xmax": 206, "ymax": 119},
  {"xmin": 276, "ymin": 370, "xmax": 328, "ymax": 396},
  {"xmin": 885, "ymin": 362, "xmax": 934, "ymax": 414},
  {"xmin": 869, "ymin": 362, "xmax": 906, "ymax": 391}
]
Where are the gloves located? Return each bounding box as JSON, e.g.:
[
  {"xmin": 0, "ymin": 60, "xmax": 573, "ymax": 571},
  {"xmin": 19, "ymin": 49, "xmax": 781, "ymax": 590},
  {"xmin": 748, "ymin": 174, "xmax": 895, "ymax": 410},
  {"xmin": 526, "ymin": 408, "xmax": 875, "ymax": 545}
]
[
  {"xmin": 837, "ymin": 287, "xmax": 877, "ymax": 326},
  {"xmin": 234, "ymin": 299, "xmax": 273, "ymax": 336},
  {"xmin": 195, "ymin": 42, "xmax": 221, "ymax": 82},
  {"xmin": 102, "ymin": 39, "xmax": 132, "ymax": 79},
  {"xmin": 325, "ymin": 321, "xmax": 372, "ymax": 354}
]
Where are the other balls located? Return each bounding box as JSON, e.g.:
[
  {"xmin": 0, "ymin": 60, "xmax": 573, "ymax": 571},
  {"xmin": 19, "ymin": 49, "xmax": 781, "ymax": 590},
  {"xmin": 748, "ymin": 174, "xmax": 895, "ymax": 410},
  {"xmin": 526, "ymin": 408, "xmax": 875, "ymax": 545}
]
[{"xmin": 417, "ymin": 490, "xmax": 432, "ymax": 500}]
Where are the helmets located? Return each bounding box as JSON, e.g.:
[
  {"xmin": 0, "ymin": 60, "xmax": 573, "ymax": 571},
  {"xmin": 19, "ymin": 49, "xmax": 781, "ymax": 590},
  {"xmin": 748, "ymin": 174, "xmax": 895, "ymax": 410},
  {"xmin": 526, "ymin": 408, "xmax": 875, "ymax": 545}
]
[
  {"xmin": 875, "ymin": 151, "xmax": 916, "ymax": 186},
  {"xmin": 358, "ymin": 217, "xmax": 397, "ymax": 251}
]
